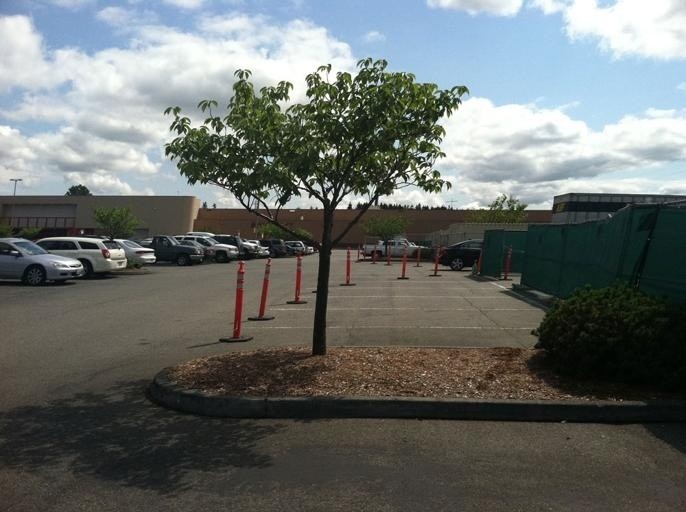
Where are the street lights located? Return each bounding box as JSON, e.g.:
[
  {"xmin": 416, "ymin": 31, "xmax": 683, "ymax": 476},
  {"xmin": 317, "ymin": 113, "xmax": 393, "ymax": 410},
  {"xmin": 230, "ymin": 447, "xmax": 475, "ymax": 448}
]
[{"xmin": 9, "ymin": 177, "xmax": 22, "ymax": 197}]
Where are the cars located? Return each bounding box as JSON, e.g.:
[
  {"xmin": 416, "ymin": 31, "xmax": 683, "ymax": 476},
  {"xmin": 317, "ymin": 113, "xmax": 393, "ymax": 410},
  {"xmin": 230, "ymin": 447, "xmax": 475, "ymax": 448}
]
[
  {"xmin": 139, "ymin": 229, "xmax": 314, "ymax": 267},
  {"xmin": 434, "ymin": 236, "xmax": 484, "ymax": 272},
  {"xmin": 0, "ymin": 236, "xmax": 86, "ymax": 288},
  {"xmin": 109, "ymin": 234, "xmax": 157, "ymax": 271},
  {"xmin": 358, "ymin": 237, "xmax": 431, "ymax": 258}
]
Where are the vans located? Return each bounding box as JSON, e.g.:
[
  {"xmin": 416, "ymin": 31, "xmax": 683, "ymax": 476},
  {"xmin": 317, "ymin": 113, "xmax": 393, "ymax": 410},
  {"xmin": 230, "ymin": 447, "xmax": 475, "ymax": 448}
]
[{"xmin": 28, "ymin": 233, "xmax": 126, "ymax": 276}]
